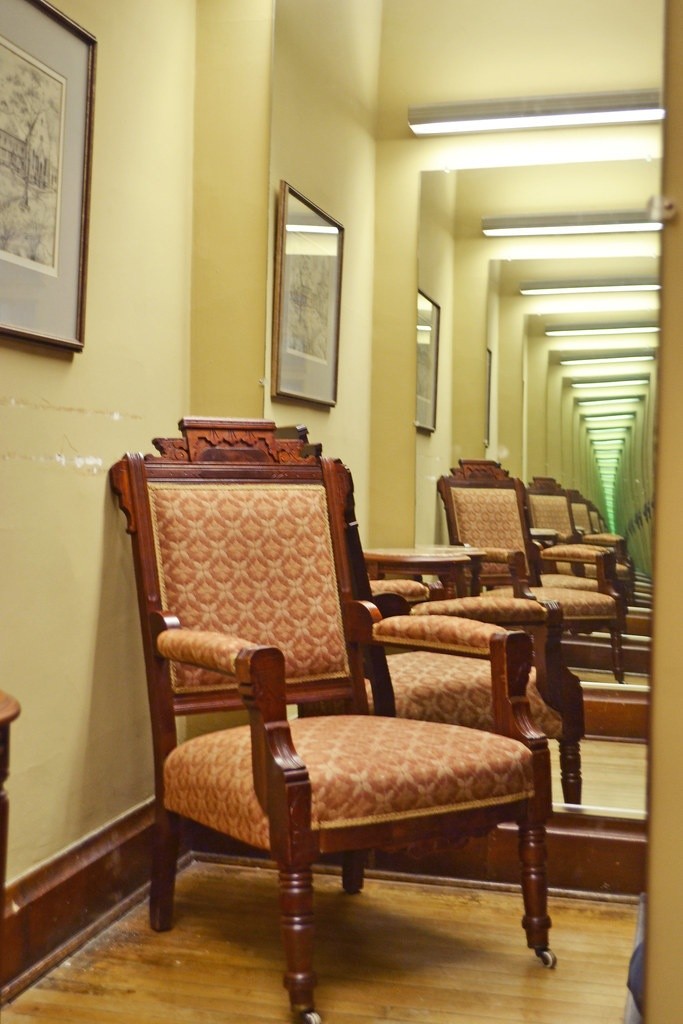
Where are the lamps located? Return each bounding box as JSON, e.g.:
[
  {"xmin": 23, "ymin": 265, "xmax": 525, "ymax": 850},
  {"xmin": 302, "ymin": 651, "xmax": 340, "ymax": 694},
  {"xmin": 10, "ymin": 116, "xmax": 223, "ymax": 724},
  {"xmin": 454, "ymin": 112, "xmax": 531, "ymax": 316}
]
[{"xmin": 400, "ymin": 97, "xmax": 667, "ymax": 405}]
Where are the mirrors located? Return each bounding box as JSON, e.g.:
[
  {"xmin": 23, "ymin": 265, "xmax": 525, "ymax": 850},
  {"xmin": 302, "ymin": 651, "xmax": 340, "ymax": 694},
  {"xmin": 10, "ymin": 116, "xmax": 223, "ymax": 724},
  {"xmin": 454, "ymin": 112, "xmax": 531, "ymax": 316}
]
[{"xmin": 269, "ymin": 0, "xmax": 669, "ymax": 824}]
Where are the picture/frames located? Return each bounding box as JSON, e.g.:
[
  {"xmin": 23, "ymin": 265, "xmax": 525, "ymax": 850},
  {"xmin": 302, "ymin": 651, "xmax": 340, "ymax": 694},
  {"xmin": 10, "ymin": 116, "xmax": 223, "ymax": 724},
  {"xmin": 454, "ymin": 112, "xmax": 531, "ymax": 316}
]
[
  {"xmin": 416, "ymin": 289, "xmax": 440, "ymax": 434},
  {"xmin": 0, "ymin": 0, "xmax": 98, "ymax": 351},
  {"xmin": 273, "ymin": 181, "xmax": 344, "ymax": 407}
]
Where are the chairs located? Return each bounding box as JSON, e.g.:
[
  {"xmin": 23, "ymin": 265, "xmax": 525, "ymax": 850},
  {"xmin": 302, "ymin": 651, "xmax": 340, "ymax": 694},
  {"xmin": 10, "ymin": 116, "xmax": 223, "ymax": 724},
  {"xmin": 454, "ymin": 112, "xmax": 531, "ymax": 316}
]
[
  {"xmin": 340, "ymin": 463, "xmax": 637, "ymax": 807},
  {"xmin": 108, "ymin": 416, "xmax": 558, "ymax": 1024}
]
